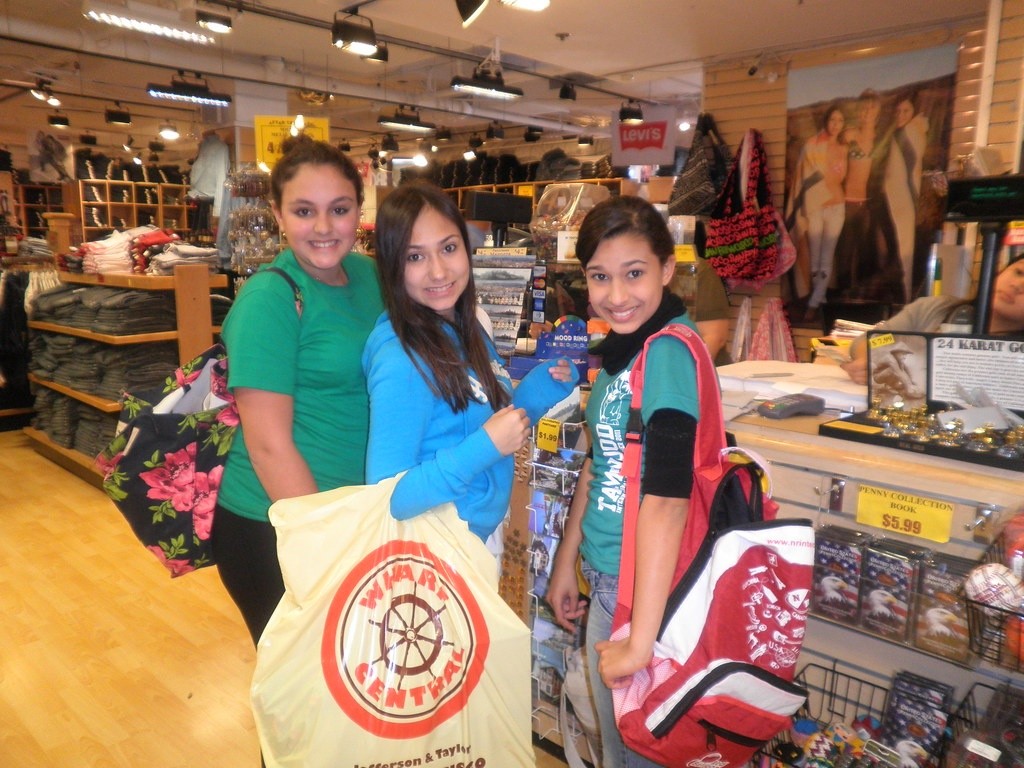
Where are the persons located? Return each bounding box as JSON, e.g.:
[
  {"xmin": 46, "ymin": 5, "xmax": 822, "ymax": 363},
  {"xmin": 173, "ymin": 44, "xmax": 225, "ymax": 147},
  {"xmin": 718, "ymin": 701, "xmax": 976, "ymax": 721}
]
[
  {"xmin": 362, "ymin": 184, "xmax": 580, "ymax": 543},
  {"xmin": 842, "ymin": 254, "xmax": 1023, "ymax": 387},
  {"xmin": 784, "ymin": 87, "xmax": 929, "ymax": 321},
  {"xmin": 546, "ymin": 194, "xmax": 726, "ymax": 768},
  {"xmin": 872, "ymin": 342, "xmax": 924, "ymax": 399},
  {"xmin": 208, "ymin": 133, "xmax": 385, "ymax": 768}
]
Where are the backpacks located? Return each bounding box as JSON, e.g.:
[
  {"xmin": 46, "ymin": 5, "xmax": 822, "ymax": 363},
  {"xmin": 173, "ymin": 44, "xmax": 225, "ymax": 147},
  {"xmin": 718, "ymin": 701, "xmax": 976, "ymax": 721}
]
[{"xmin": 608, "ymin": 324, "xmax": 811, "ymax": 768}]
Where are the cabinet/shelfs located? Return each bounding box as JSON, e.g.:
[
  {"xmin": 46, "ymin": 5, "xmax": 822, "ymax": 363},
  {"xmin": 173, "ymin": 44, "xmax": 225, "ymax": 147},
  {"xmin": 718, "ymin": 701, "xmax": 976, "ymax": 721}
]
[
  {"xmin": 441, "ymin": 179, "xmax": 639, "ymax": 216},
  {"xmin": 0, "ymin": 179, "xmax": 228, "ymax": 480}
]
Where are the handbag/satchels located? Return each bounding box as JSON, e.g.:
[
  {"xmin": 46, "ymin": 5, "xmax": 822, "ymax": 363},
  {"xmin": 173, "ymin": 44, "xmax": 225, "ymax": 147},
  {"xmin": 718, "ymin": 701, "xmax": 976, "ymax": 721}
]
[
  {"xmin": 92, "ymin": 266, "xmax": 304, "ymax": 578},
  {"xmin": 667, "ymin": 112, "xmax": 798, "ymax": 291},
  {"xmin": 250, "ymin": 470, "xmax": 535, "ymax": 768},
  {"xmin": 559, "ymin": 644, "xmax": 601, "ymax": 768}
]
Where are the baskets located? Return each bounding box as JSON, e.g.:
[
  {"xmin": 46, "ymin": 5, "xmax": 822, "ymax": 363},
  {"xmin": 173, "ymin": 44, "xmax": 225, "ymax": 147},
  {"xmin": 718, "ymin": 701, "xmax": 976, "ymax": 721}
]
[
  {"xmin": 960, "ymin": 595, "xmax": 1024, "ymax": 672},
  {"xmin": 936, "ymin": 682, "xmax": 997, "ymax": 768},
  {"xmin": 751, "ymin": 663, "xmax": 891, "ymax": 768}
]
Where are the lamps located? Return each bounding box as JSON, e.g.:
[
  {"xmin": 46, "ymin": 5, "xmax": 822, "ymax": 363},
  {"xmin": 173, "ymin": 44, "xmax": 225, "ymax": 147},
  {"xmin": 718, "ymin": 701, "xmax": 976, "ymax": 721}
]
[
  {"xmin": 360, "ymin": 42, "xmax": 388, "ymax": 64},
  {"xmin": 148, "ymin": 152, "xmax": 159, "ymax": 161},
  {"xmin": 30, "ymin": 81, "xmax": 49, "ymax": 100},
  {"xmin": 79, "ymin": 130, "xmax": 97, "ymax": 146},
  {"xmin": 47, "ymin": 90, "xmax": 61, "ymax": 105},
  {"xmin": 559, "ymin": 84, "xmax": 577, "ymax": 100},
  {"xmin": 158, "ymin": 119, "xmax": 181, "ymax": 141},
  {"xmin": 47, "ymin": 110, "xmax": 69, "ymax": 128},
  {"xmin": 196, "ymin": 10, "xmax": 233, "ymax": 33},
  {"xmin": 619, "ymin": 98, "xmax": 644, "ymax": 124},
  {"xmin": 338, "ymin": 106, "xmax": 594, "ymax": 173},
  {"xmin": 104, "ymin": 102, "xmax": 131, "ymax": 124},
  {"xmin": 148, "ymin": 139, "xmax": 164, "ymax": 150},
  {"xmin": 144, "ymin": 71, "xmax": 232, "ymax": 108},
  {"xmin": 329, "ymin": 0, "xmax": 390, "ymax": 56},
  {"xmin": 455, "ymin": 0, "xmax": 488, "ymax": 29},
  {"xmin": 133, "ymin": 152, "xmax": 142, "ymax": 163},
  {"xmin": 450, "ymin": 65, "xmax": 524, "ymax": 99},
  {"xmin": 122, "ymin": 137, "xmax": 134, "ymax": 151}
]
